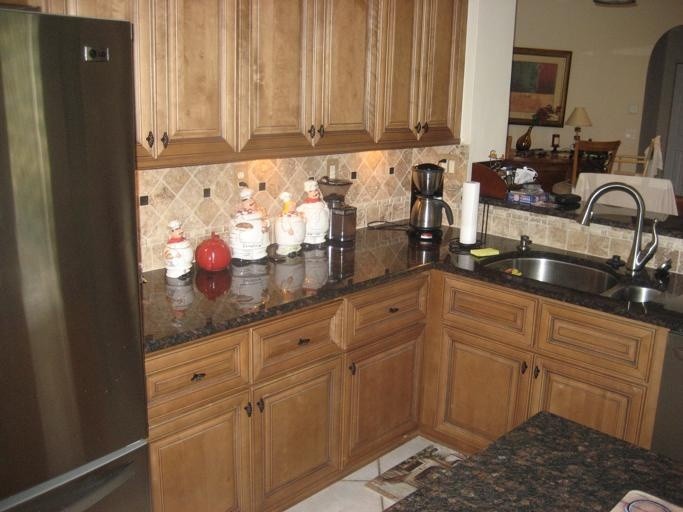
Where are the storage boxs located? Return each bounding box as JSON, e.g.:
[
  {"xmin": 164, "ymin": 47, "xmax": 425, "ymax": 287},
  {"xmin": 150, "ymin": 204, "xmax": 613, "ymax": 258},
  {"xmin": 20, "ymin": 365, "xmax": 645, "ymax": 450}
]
[{"xmin": 472, "ymin": 159, "xmax": 540, "ymax": 199}]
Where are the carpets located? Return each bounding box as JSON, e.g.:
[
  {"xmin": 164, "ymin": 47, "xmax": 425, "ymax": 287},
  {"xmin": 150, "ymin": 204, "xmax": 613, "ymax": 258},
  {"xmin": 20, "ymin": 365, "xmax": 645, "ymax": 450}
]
[{"xmin": 364, "ymin": 443, "xmax": 468, "ymax": 502}]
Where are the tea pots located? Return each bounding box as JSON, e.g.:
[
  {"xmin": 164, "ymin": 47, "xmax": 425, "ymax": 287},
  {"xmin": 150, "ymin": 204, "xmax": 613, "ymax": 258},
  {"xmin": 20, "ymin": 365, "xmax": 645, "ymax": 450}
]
[
  {"xmin": 196, "ymin": 231, "xmax": 231, "ymax": 273},
  {"xmin": 194, "ymin": 269, "xmax": 232, "ymax": 303}
]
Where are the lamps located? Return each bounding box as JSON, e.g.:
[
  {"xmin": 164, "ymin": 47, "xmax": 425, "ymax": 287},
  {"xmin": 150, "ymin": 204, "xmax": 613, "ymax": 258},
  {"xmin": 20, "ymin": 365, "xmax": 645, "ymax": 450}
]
[{"xmin": 566, "ymin": 107, "xmax": 592, "ymax": 197}]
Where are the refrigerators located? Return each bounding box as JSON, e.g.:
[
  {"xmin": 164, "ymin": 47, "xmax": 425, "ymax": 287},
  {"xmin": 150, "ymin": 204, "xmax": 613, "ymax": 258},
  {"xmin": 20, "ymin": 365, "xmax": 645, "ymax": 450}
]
[{"xmin": 0, "ymin": 8, "xmax": 153, "ymax": 512}]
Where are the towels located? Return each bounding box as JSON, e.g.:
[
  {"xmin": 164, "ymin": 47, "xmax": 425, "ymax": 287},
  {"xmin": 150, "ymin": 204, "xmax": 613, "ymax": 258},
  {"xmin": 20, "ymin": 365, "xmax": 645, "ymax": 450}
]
[{"xmin": 643, "ymin": 136, "xmax": 663, "ymax": 177}]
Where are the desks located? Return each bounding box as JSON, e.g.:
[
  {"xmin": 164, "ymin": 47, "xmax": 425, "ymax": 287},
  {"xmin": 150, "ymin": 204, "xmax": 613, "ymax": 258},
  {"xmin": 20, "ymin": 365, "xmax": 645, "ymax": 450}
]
[{"xmin": 509, "ymin": 147, "xmax": 605, "ymax": 193}]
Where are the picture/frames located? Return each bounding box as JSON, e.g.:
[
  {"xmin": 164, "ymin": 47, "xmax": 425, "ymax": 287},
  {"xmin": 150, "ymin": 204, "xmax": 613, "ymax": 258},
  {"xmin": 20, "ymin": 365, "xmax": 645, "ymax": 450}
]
[{"xmin": 508, "ymin": 47, "xmax": 573, "ymax": 128}]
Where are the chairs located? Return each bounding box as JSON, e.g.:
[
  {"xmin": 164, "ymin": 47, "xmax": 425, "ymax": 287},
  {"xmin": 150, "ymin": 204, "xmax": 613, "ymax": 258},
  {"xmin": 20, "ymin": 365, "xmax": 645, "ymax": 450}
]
[
  {"xmin": 552, "ymin": 140, "xmax": 621, "ymax": 194},
  {"xmin": 612, "ymin": 135, "xmax": 662, "ymax": 178}
]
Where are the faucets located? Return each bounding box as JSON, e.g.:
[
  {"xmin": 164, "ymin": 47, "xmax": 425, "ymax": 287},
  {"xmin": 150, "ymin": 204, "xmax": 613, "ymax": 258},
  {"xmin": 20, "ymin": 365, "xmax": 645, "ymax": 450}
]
[{"xmin": 579, "ymin": 182, "xmax": 658, "ymax": 271}]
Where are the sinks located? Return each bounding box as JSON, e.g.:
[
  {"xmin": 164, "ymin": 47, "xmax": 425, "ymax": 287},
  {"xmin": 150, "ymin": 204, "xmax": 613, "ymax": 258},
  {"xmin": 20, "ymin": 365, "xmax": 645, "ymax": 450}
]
[
  {"xmin": 483, "ymin": 258, "xmax": 616, "ymax": 294},
  {"xmin": 614, "ymin": 286, "xmax": 659, "ymax": 303}
]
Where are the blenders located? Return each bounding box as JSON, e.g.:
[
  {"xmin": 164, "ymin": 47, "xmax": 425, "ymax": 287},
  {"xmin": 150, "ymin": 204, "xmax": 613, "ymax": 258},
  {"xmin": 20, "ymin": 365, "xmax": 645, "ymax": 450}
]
[
  {"xmin": 328, "ymin": 243, "xmax": 356, "ymax": 280},
  {"xmin": 319, "ymin": 177, "xmax": 356, "ymax": 247}
]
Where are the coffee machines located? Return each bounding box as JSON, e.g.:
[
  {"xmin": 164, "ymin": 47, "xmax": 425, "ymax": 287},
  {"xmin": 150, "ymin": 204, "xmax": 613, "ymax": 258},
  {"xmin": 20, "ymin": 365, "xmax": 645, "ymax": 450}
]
[
  {"xmin": 407, "ymin": 240, "xmax": 439, "ymax": 266},
  {"xmin": 408, "ymin": 163, "xmax": 453, "ymax": 239}
]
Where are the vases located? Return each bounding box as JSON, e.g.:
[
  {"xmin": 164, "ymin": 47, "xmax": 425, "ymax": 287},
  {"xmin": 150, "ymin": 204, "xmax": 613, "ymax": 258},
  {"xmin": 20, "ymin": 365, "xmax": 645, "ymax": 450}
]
[{"xmin": 516, "ymin": 128, "xmax": 535, "ymax": 150}]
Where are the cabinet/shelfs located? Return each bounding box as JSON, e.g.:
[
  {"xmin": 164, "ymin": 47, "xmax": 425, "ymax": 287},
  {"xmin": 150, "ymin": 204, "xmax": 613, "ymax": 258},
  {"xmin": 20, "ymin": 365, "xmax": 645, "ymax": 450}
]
[
  {"xmin": 70, "ymin": 0, "xmax": 238, "ymax": 169},
  {"xmin": 239, "ymin": 0, "xmax": 375, "ymax": 162},
  {"xmin": 417, "ymin": 270, "xmax": 670, "ymax": 452},
  {"xmin": 344, "ymin": 273, "xmax": 430, "ymax": 477},
  {"xmin": 379, "ymin": 1, "xmax": 468, "ymax": 151},
  {"xmin": 145, "ymin": 299, "xmax": 344, "ymax": 512}
]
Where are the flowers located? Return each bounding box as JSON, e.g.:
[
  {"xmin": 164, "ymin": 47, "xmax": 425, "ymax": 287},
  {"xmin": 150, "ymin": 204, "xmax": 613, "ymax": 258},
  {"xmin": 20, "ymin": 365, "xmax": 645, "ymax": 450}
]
[{"xmin": 529, "ymin": 105, "xmax": 562, "ymax": 128}]
